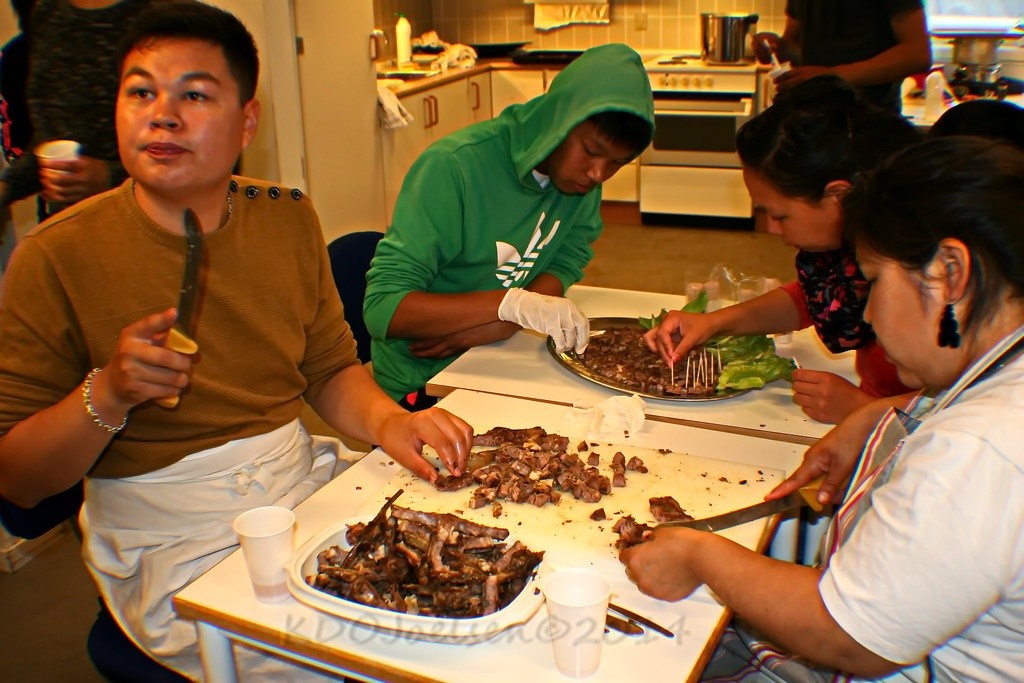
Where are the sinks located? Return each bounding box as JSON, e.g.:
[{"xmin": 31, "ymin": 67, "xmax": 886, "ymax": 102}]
[{"xmin": 377, "ymin": 70, "xmax": 442, "ymax": 82}]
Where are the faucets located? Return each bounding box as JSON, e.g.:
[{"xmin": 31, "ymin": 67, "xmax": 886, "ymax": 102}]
[{"xmin": 374, "ymin": 28, "xmax": 388, "ymax": 45}]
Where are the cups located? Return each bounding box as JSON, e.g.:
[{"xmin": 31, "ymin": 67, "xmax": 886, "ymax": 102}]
[
  {"xmin": 34, "ymin": 139, "xmax": 81, "ymax": 175},
  {"xmin": 233, "ymin": 506, "xmax": 295, "ymax": 604},
  {"xmin": 768, "ymin": 61, "xmax": 792, "ymax": 94},
  {"xmin": 540, "ymin": 572, "xmax": 611, "ymax": 677}
]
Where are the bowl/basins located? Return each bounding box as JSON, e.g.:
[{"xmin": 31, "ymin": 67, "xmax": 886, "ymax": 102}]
[{"xmin": 467, "ymin": 40, "xmax": 531, "ymax": 58}]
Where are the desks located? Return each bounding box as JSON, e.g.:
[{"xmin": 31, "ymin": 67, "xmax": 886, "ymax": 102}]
[
  {"xmin": 172, "ymin": 388, "xmax": 814, "ymax": 683},
  {"xmin": 426, "ymin": 284, "xmax": 860, "ymax": 447}
]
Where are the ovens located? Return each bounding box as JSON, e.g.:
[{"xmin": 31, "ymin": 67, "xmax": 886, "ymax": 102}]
[{"xmin": 638, "ymin": 95, "xmax": 760, "ymax": 229}]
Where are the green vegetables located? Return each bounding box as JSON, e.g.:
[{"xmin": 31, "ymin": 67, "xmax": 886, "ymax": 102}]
[{"xmin": 640, "ymin": 290, "xmax": 799, "ymax": 389}]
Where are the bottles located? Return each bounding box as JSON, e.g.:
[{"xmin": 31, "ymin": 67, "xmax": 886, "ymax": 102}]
[{"xmin": 395, "ymin": 12, "xmax": 411, "ymax": 69}]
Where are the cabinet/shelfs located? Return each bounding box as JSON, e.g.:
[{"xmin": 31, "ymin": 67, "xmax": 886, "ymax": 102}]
[{"xmin": 293, "ymin": 0, "xmax": 639, "ymax": 245}]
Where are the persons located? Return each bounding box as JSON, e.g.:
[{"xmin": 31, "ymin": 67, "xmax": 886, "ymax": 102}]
[
  {"xmin": 639, "ymin": 72, "xmax": 931, "ymax": 395},
  {"xmin": 616, "ymin": 140, "xmax": 1022, "ymax": 683},
  {"xmin": 751, "ymin": 0, "xmax": 934, "ymax": 116},
  {"xmin": 0, "ymin": 0, "xmax": 471, "ymax": 683},
  {"xmin": 361, "ymin": 43, "xmax": 653, "ymax": 412}
]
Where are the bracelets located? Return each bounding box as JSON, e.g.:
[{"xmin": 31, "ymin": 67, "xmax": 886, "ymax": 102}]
[{"xmin": 81, "ymin": 368, "xmax": 128, "ymax": 433}]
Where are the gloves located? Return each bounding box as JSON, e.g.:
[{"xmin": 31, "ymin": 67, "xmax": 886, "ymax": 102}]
[
  {"xmin": 499, "ymin": 287, "xmax": 591, "ymax": 356},
  {"xmin": 565, "ymin": 392, "xmax": 648, "ymax": 439}
]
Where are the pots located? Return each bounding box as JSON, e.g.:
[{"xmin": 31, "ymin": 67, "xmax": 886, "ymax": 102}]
[{"xmin": 699, "ymin": 13, "xmax": 759, "ymax": 65}]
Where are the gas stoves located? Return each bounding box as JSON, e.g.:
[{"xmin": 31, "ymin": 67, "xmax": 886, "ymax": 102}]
[{"xmin": 640, "ymin": 46, "xmax": 760, "ymax": 94}]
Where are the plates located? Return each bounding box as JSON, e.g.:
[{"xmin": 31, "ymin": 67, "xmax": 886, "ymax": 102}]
[{"xmin": 286, "ymin": 509, "xmax": 545, "ymax": 636}]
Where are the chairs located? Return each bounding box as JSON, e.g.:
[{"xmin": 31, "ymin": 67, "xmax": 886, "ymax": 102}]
[
  {"xmin": 325, "ymin": 231, "xmax": 385, "ymax": 364},
  {"xmin": 0, "ymin": 479, "xmax": 193, "ymax": 683}
]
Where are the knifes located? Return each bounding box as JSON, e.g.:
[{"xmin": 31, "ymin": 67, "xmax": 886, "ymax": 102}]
[
  {"xmin": 654, "ymin": 472, "xmax": 839, "ymax": 540},
  {"xmin": 153, "ymin": 206, "xmax": 201, "ymax": 409}
]
